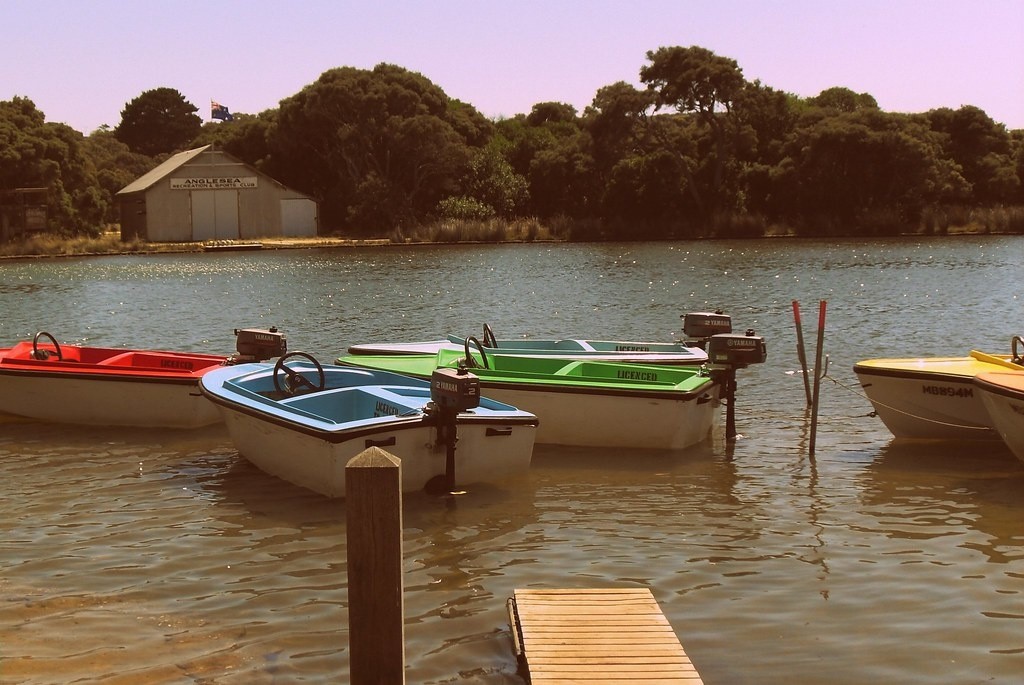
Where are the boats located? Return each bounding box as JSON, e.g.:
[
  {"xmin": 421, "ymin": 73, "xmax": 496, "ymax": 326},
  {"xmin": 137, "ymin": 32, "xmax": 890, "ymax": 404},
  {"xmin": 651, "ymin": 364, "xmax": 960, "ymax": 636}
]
[
  {"xmin": 334, "ymin": 327, "xmax": 774, "ymax": 453},
  {"xmin": 201, "ymin": 349, "xmax": 540, "ymax": 502},
  {"xmin": 0, "ymin": 325, "xmax": 293, "ymax": 433},
  {"xmin": 345, "ymin": 307, "xmax": 734, "ymax": 362},
  {"xmin": 972, "ymin": 371, "xmax": 1024, "ymax": 465},
  {"xmin": 853, "ymin": 335, "xmax": 1024, "ymax": 443},
  {"xmin": 205, "ymin": 242, "xmax": 264, "ymax": 251}
]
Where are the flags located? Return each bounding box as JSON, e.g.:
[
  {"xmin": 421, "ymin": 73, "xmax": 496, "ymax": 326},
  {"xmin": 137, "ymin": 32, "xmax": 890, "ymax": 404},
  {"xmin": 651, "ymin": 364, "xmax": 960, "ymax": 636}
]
[{"xmin": 212, "ymin": 102, "xmax": 232, "ymax": 121}]
